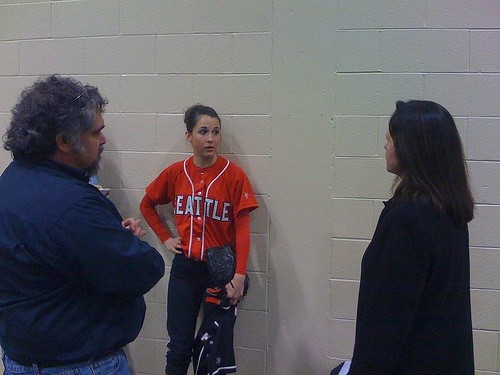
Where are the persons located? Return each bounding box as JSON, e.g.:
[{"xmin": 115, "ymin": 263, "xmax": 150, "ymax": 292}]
[
  {"xmin": 140, "ymin": 105, "xmax": 259, "ymax": 375},
  {"xmin": 0, "ymin": 74, "xmax": 164, "ymax": 375},
  {"xmin": 347, "ymin": 99, "xmax": 476, "ymax": 375}
]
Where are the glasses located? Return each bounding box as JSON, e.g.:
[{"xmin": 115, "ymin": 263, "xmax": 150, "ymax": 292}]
[{"xmin": 72, "ymin": 81, "xmax": 88, "ymax": 104}]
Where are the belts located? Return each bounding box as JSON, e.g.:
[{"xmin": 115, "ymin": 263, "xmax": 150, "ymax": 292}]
[{"xmin": 5, "ymin": 348, "xmax": 113, "ymax": 368}]
